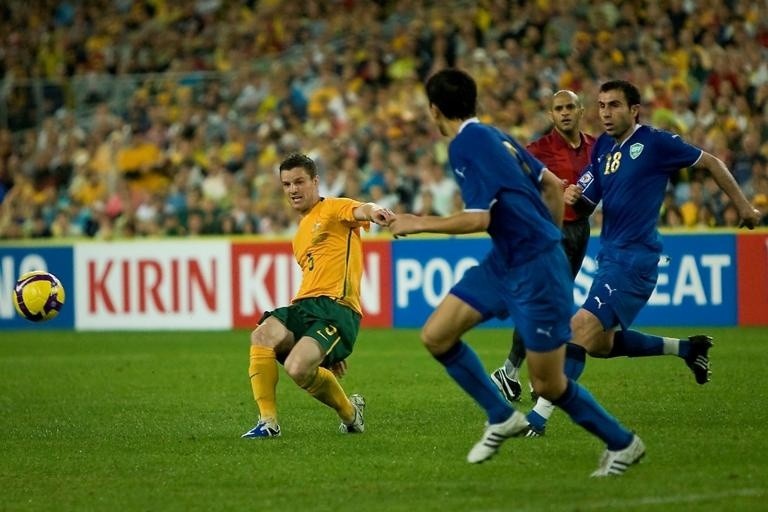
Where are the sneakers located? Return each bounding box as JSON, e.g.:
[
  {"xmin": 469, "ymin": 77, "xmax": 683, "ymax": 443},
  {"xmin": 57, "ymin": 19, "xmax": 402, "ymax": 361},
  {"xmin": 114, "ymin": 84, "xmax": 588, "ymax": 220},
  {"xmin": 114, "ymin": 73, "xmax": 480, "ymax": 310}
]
[
  {"xmin": 241, "ymin": 419, "xmax": 282, "ymax": 439},
  {"xmin": 467, "ymin": 409, "xmax": 545, "ymax": 464},
  {"xmin": 589, "ymin": 430, "xmax": 647, "ymax": 476},
  {"xmin": 489, "ymin": 364, "xmax": 522, "ymax": 405},
  {"xmin": 343, "ymin": 392, "xmax": 366, "ymax": 433},
  {"xmin": 685, "ymin": 335, "xmax": 714, "ymax": 384}
]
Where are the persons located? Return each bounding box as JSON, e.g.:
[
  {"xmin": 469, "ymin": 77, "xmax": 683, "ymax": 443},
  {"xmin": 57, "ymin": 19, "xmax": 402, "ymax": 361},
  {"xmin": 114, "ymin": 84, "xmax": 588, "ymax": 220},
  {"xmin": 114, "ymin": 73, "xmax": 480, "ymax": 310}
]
[
  {"xmin": 512, "ymin": 81, "xmax": 762, "ymax": 438},
  {"xmin": 491, "ymin": 90, "xmax": 597, "ymax": 402},
  {"xmin": 387, "ymin": 68, "xmax": 647, "ymax": 476},
  {"xmin": 241, "ymin": 153, "xmax": 396, "ymax": 438}
]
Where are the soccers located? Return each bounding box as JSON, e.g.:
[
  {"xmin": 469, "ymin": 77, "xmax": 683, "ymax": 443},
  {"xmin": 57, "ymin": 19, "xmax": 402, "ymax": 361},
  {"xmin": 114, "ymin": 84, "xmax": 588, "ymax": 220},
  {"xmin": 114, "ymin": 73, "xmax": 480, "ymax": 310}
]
[{"xmin": 13, "ymin": 271, "xmax": 65, "ymax": 322}]
